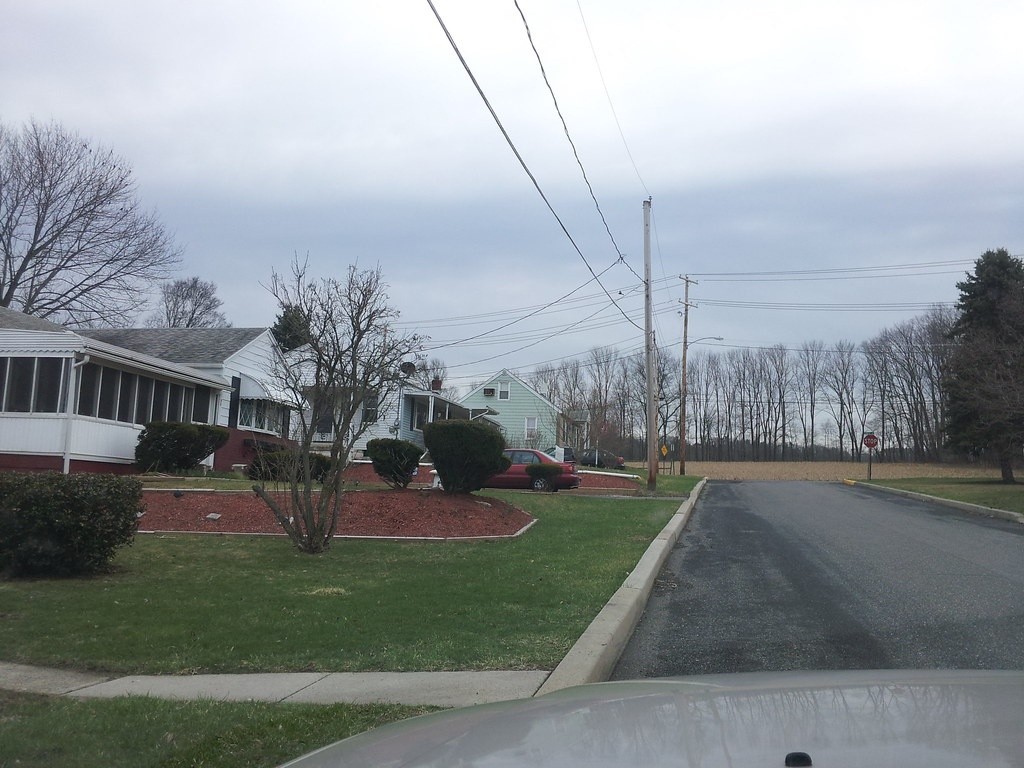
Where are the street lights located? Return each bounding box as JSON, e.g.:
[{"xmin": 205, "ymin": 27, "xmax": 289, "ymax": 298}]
[{"xmin": 680, "ymin": 336, "xmax": 724, "ymax": 475}]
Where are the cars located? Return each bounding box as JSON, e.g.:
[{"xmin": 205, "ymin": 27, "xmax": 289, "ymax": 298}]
[
  {"xmin": 480, "ymin": 448, "xmax": 582, "ymax": 493},
  {"xmin": 576, "ymin": 447, "xmax": 626, "ymax": 471}
]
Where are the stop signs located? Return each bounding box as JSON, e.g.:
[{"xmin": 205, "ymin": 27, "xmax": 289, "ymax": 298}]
[{"xmin": 864, "ymin": 434, "xmax": 878, "ymax": 448}]
